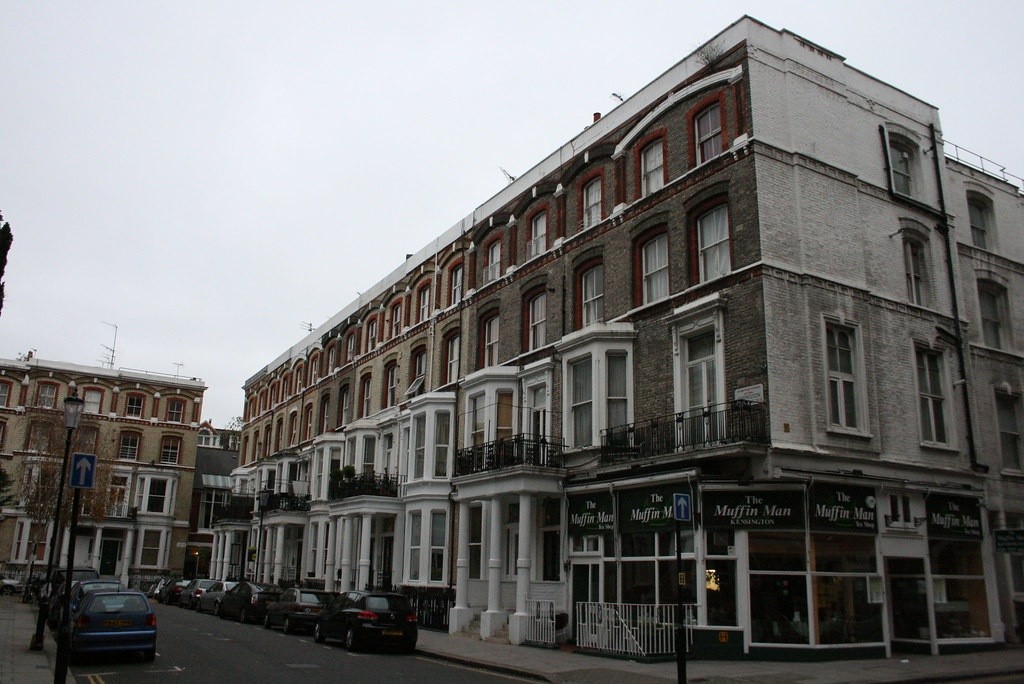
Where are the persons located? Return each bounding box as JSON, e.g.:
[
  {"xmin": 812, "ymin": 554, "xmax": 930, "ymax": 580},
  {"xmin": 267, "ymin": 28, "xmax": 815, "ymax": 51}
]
[{"xmin": 46, "ymin": 568, "xmax": 67, "ymax": 632}]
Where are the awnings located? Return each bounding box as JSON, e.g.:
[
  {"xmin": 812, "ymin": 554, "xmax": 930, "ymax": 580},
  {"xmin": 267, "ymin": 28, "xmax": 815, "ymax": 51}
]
[{"xmin": 202, "ymin": 474, "xmax": 234, "ymax": 490}]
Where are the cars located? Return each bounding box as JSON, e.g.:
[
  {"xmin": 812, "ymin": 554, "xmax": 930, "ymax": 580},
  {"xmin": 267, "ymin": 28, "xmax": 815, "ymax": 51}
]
[
  {"xmin": 312, "ymin": 591, "xmax": 418, "ymax": 653},
  {"xmin": 263, "ymin": 587, "xmax": 333, "ymax": 634},
  {"xmin": 70, "ymin": 587, "xmax": 157, "ymax": 665},
  {"xmin": 68, "ymin": 580, "xmax": 128, "ymax": 622},
  {"xmin": 46, "ymin": 579, "xmax": 83, "ymax": 618},
  {"xmin": 51, "ymin": 567, "xmax": 100, "ymax": 587},
  {"xmin": 218, "ymin": 580, "xmax": 285, "ymax": 624},
  {"xmin": 194, "ymin": 581, "xmax": 241, "ymax": 615},
  {"xmin": 0, "ymin": 570, "xmax": 23, "ymax": 597},
  {"xmin": 177, "ymin": 578, "xmax": 215, "ymax": 611},
  {"xmin": 156, "ymin": 578, "xmax": 193, "ymax": 605}
]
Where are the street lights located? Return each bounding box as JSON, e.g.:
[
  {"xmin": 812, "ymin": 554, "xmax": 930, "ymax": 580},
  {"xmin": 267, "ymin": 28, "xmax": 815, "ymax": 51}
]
[
  {"xmin": 27, "ymin": 385, "xmax": 86, "ymax": 652},
  {"xmin": 254, "ymin": 484, "xmax": 271, "ymax": 582}
]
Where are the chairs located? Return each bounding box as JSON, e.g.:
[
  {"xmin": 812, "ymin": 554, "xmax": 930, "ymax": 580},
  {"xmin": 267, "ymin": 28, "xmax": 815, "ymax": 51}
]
[
  {"xmin": 92, "ymin": 600, "xmax": 106, "ymax": 613},
  {"xmin": 606, "ymin": 428, "xmax": 647, "ymax": 463},
  {"xmin": 117, "ymin": 599, "xmax": 140, "ymax": 612}
]
